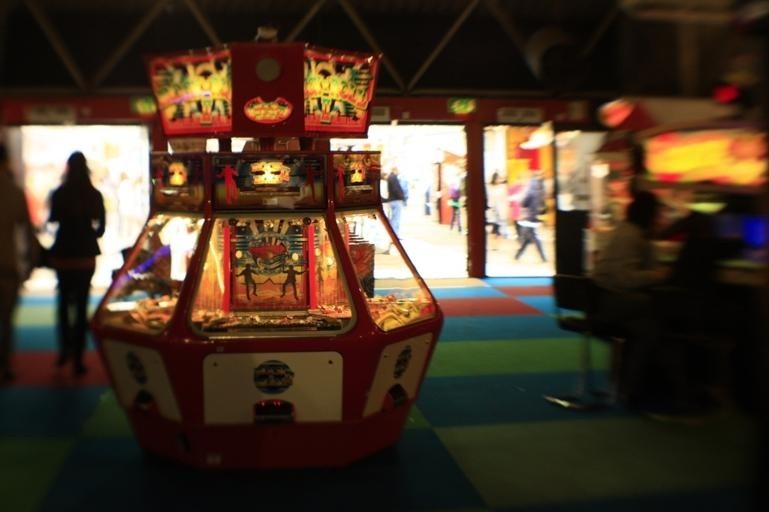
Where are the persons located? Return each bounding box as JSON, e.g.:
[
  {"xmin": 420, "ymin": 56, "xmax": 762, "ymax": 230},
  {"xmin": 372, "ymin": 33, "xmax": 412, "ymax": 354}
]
[
  {"xmin": 0, "ymin": 142, "xmax": 43, "ymax": 381},
  {"xmin": 659, "ymin": 191, "xmax": 759, "ymax": 309},
  {"xmin": 378, "ymin": 167, "xmax": 410, "ymax": 242},
  {"xmin": 46, "ymin": 151, "xmax": 106, "ymax": 376},
  {"xmin": 448, "ymin": 169, "xmax": 552, "ymax": 261},
  {"xmin": 600, "ymin": 186, "xmax": 673, "ymax": 292}
]
